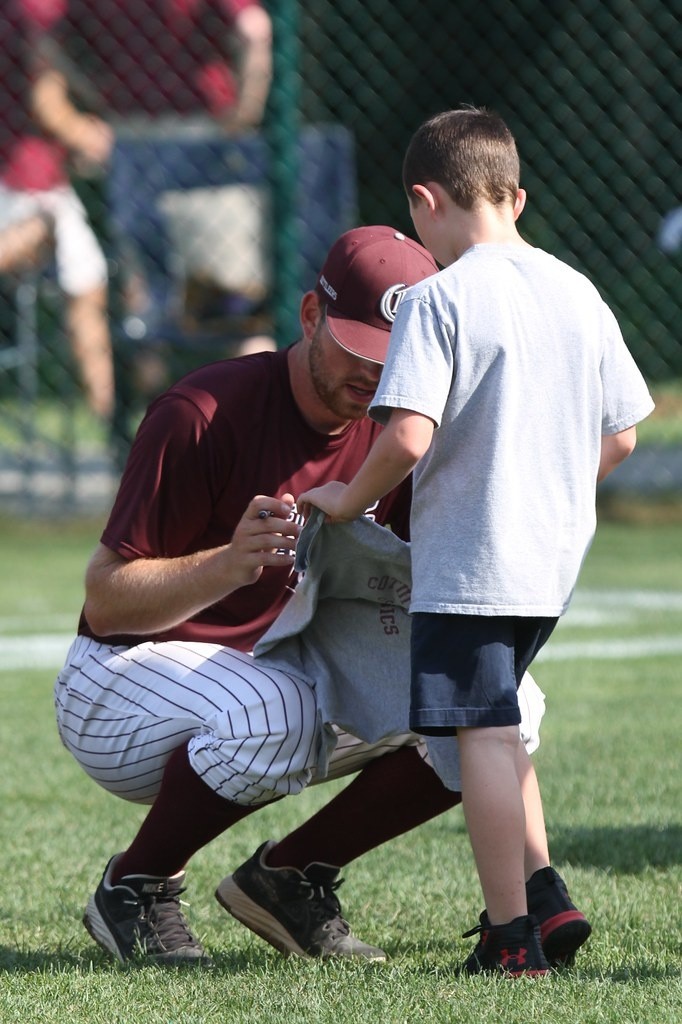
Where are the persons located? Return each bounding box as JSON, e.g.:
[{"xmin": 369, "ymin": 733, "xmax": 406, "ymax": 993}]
[
  {"xmin": 54, "ymin": 223, "xmax": 547, "ymax": 966},
  {"xmin": 0, "ymin": 0, "xmax": 277, "ymax": 414},
  {"xmin": 296, "ymin": 105, "xmax": 654, "ymax": 975}
]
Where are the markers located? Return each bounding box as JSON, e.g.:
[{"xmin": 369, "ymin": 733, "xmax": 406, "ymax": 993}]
[{"xmin": 259, "ymin": 511, "xmax": 269, "ymax": 519}]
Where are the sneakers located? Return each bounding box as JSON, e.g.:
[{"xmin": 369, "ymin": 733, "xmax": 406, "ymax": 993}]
[
  {"xmin": 216, "ymin": 840, "xmax": 390, "ymax": 966},
  {"xmin": 525, "ymin": 866, "xmax": 592, "ymax": 970},
  {"xmin": 451, "ymin": 909, "xmax": 552, "ymax": 977},
  {"xmin": 80, "ymin": 853, "xmax": 215, "ymax": 968}
]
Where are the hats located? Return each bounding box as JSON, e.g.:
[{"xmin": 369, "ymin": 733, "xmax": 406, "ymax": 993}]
[{"xmin": 318, "ymin": 226, "xmax": 439, "ymax": 365}]
